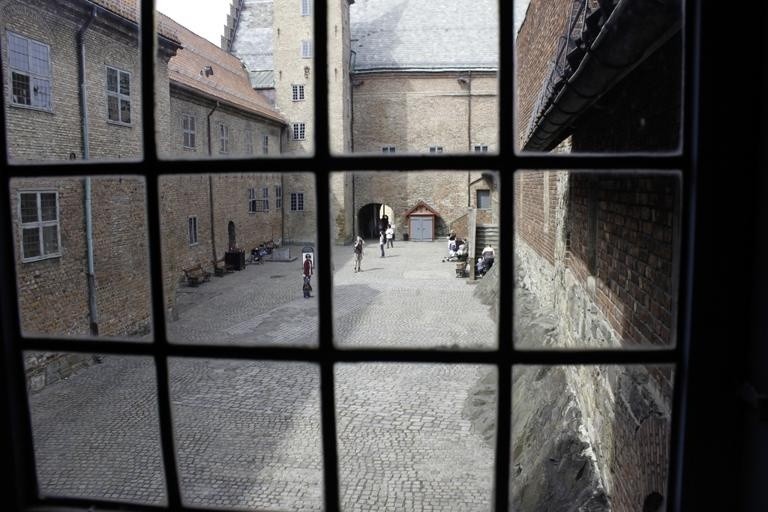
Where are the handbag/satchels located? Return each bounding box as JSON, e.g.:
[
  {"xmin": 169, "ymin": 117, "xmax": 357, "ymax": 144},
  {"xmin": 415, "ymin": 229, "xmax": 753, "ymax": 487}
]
[{"xmin": 304, "ymin": 283, "xmax": 312, "ymax": 291}]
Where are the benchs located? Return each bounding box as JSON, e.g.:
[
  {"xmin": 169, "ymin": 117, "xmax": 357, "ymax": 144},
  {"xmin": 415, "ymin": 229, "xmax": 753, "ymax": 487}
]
[{"xmin": 183, "ymin": 258, "xmax": 234, "ymax": 288}]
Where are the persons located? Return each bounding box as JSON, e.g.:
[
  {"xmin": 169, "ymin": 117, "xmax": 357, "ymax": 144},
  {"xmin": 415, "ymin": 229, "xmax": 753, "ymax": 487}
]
[
  {"xmin": 352, "ymin": 235, "xmax": 365, "ymax": 272},
  {"xmin": 384, "ymin": 223, "xmax": 394, "ymax": 248},
  {"xmin": 378, "ymin": 230, "xmax": 386, "ymax": 257},
  {"xmin": 482, "ymin": 244, "xmax": 495, "ymax": 273},
  {"xmin": 475, "ymin": 257, "xmax": 485, "ymax": 278},
  {"xmin": 301, "ymin": 253, "xmax": 312, "ymax": 299},
  {"xmin": 377, "ymin": 215, "xmax": 391, "ymax": 232},
  {"xmin": 445, "ymin": 229, "xmax": 468, "ymax": 262}
]
